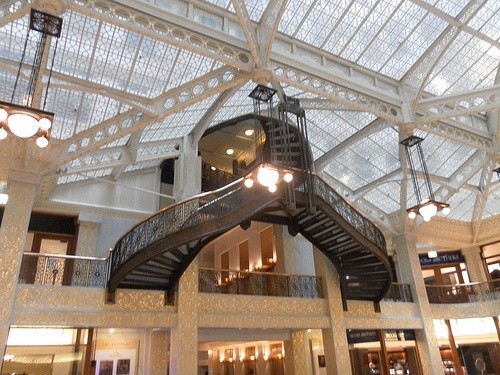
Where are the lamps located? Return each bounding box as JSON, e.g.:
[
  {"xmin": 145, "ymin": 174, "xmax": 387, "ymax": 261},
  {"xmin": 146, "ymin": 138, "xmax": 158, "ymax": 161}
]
[
  {"xmin": 406, "ymin": 198, "xmax": 450, "ymax": 222},
  {"xmin": 215, "ymin": 258, "xmax": 273, "ymax": 288},
  {"xmin": 243, "ymin": 161, "xmax": 294, "ymax": 193},
  {"xmin": 219, "ymin": 353, "xmax": 282, "ymax": 363},
  {"xmin": 0, "ymin": 100, "xmax": 55, "ymax": 148},
  {"xmin": 428, "ymin": 251, "xmax": 437, "ymax": 258}
]
